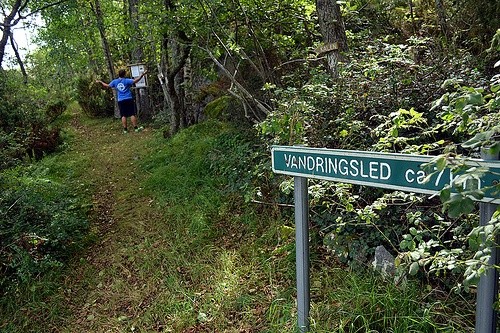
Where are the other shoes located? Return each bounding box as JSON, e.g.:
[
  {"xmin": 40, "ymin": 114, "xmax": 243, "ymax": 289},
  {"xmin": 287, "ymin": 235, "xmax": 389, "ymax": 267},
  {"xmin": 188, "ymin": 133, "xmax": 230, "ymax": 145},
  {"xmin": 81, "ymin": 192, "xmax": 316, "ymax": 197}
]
[
  {"xmin": 124, "ymin": 130, "xmax": 129, "ymax": 134},
  {"xmin": 134, "ymin": 128, "xmax": 142, "ymax": 132}
]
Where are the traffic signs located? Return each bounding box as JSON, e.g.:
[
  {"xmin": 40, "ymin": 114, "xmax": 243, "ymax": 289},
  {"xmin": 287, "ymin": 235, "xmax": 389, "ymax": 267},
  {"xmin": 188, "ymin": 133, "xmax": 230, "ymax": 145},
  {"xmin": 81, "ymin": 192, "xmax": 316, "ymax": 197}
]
[{"xmin": 270, "ymin": 144, "xmax": 500, "ymax": 205}]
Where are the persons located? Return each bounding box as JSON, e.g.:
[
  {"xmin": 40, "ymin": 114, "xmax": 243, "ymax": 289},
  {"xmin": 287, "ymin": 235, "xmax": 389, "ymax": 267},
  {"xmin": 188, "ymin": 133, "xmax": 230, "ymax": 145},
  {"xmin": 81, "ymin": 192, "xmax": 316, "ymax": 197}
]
[{"xmin": 95, "ymin": 68, "xmax": 149, "ymax": 132}]
